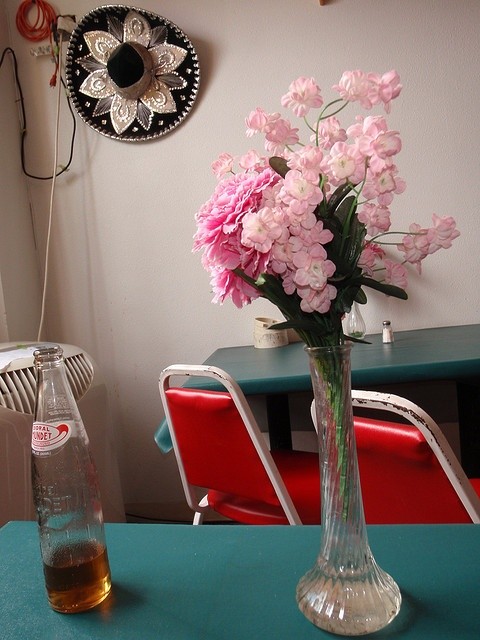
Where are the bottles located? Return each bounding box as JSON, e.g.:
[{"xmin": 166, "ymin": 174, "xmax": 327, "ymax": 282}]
[
  {"xmin": 382, "ymin": 320, "xmax": 394, "ymax": 343},
  {"xmin": 33, "ymin": 345, "xmax": 112, "ymax": 614}
]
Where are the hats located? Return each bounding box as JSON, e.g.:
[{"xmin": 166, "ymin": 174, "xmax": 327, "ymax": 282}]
[{"xmin": 65, "ymin": 4, "xmax": 201, "ymax": 143}]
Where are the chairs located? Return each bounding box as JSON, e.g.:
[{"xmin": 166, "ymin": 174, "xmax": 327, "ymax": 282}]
[
  {"xmin": 310, "ymin": 388, "xmax": 480, "ymax": 523},
  {"xmin": 156, "ymin": 363, "xmax": 323, "ymax": 524}
]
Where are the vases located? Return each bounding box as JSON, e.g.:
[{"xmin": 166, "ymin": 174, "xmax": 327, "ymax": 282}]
[{"xmin": 295, "ymin": 343, "xmax": 403, "ymax": 637}]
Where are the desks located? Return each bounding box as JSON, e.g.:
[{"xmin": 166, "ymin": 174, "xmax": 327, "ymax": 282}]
[
  {"xmin": 1, "ymin": 521, "xmax": 479, "ymax": 640},
  {"xmin": 150, "ymin": 322, "xmax": 480, "ymax": 480}
]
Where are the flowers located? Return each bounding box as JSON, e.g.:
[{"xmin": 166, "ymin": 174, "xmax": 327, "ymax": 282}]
[{"xmin": 192, "ymin": 66, "xmax": 464, "ymax": 522}]
